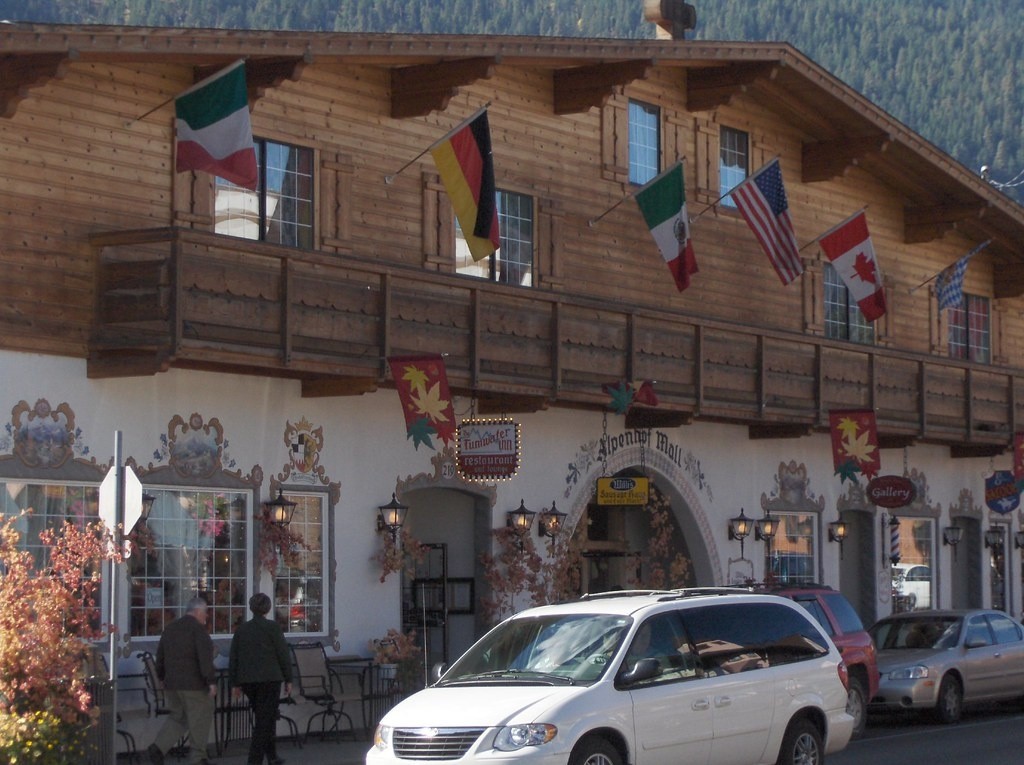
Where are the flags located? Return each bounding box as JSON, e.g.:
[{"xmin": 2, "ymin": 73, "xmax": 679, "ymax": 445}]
[
  {"xmin": 818, "ymin": 209, "xmax": 886, "ymax": 323},
  {"xmin": 935, "ymin": 240, "xmax": 991, "ymax": 317},
  {"xmin": 174, "ymin": 59, "xmax": 258, "ymax": 192},
  {"xmin": 429, "ymin": 104, "xmax": 500, "ymax": 262},
  {"xmin": 729, "ymin": 156, "xmax": 803, "ymax": 287},
  {"xmin": 631, "ymin": 159, "xmax": 699, "ymax": 293}
]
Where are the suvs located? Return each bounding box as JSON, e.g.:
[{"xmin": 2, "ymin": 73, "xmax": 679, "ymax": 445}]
[
  {"xmin": 726, "ymin": 584, "xmax": 880, "ymax": 740},
  {"xmin": 365, "ymin": 586, "xmax": 855, "ymax": 765}
]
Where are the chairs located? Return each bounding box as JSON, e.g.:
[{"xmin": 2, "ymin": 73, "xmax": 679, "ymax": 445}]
[
  {"xmin": 75, "ymin": 654, "xmax": 140, "ymax": 765},
  {"xmin": 290, "ymin": 640, "xmax": 363, "ymax": 744}
]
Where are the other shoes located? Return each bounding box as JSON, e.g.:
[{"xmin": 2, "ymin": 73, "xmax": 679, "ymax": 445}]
[
  {"xmin": 148, "ymin": 744, "xmax": 164, "ymax": 765},
  {"xmin": 267, "ymin": 756, "xmax": 287, "ymax": 765}
]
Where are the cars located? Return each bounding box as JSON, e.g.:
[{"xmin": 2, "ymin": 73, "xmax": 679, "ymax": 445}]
[
  {"xmin": 274, "ymin": 576, "xmax": 322, "ymax": 628},
  {"xmin": 866, "ymin": 607, "xmax": 1023, "ymax": 727},
  {"xmin": 890, "ymin": 562, "xmax": 930, "ymax": 608}
]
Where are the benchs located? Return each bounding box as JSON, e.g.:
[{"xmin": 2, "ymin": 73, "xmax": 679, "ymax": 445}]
[{"xmin": 134, "ymin": 650, "xmax": 303, "ymax": 757}]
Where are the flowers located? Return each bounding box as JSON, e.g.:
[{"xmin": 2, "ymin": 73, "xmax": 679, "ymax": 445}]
[
  {"xmin": 72, "ymin": 497, "xmax": 88, "ymax": 525},
  {"xmin": 368, "ymin": 627, "xmax": 425, "ymax": 700},
  {"xmin": 188, "ymin": 497, "xmax": 228, "ymax": 538}
]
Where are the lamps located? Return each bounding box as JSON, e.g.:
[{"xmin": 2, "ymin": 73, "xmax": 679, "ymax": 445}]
[
  {"xmin": 828, "ymin": 511, "xmax": 852, "ymax": 560},
  {"xmin": 943, "ymin": 517, "xmax": 965, "ymax": 562},
  {"xmin": 506, "ymin": 498, "xmax": 537, "ymax": 554},
  {"xmin": 755, "ymin": 508, "xmax": 781, "ymax": 560},
  {"xmin": 265, "ymin": 487, "xmax": 299, "ymax": 528},
  {"xmin": 538, "ymin": 499, "xmax": 569, "ymax": 558},
  {"xmin": 984, "ymin": 519, "xmax": 1005, "ymax": 561},
  {"xmin": 880, "ymin": 512, "xmax": 901, "ymax": 569},
  {"xmin": 1014, "ymin": 531, "xmax": 1024, "ymax": 549},
  {"xmin": 377, "ymin": 492, "xmax": 410, "ymax": 545},
  {"xmin": 728, "ymin": 507, "xmax": 755, "ymax": 559}
]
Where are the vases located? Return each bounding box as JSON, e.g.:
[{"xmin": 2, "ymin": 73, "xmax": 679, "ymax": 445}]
[{"xmin": 377, "ymin": 663, "xmax": 398, "ymax": 679}]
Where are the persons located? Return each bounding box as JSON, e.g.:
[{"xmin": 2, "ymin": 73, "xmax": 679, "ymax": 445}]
[
  {"xmin": 588, "ymin": 621, "xmax": 671, "ymax": 675},
  {"xmin": 229, "ymin": 593, "xmax": 294, "ymax": 765},
  {"xmin": 149, "ymin": 598, "xmax": 217, "ymax": 765}
]
